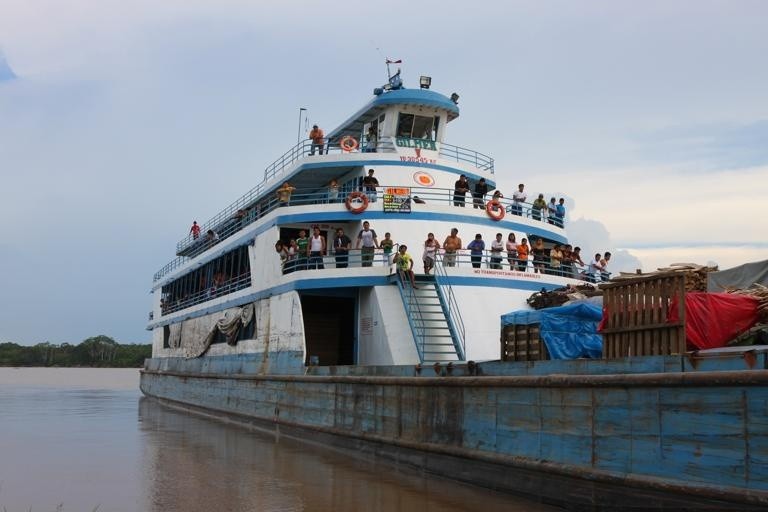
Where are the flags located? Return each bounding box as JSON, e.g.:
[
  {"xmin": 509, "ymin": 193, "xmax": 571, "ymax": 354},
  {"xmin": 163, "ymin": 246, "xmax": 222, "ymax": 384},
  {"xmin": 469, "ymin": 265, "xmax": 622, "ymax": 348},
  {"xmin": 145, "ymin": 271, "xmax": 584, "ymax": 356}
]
[{"xmin": 386, "ymin": 57, "xmax": 402, "ymax": 64}]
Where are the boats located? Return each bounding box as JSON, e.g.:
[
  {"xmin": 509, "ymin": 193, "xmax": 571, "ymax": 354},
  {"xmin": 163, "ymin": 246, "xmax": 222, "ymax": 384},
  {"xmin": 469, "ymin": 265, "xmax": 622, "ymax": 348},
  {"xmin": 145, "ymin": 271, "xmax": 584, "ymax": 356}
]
[{"xmin": 139, "ymin": 53, "xmax": 768, "ymax": 510}]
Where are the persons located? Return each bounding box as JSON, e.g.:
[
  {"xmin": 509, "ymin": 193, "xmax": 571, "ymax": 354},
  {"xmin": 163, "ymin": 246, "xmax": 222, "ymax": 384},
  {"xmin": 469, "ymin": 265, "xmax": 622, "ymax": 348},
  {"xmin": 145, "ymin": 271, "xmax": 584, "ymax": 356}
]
[
  {"xmin": 159, "ymin": 270, "xmax": 229, "ymax": 313},
  {"xmin": 588, "ymin": 252, "xmax": 600, "ymax": 280},
  {"xmin": 600, "ymin": 252, "xmax": 611, "ymax": 281},
  {"xmin": 276, "ymin": 221, "xmax": 393, "ymax": 273},
  {"xmin": 363, "ymin": 170, "xmax": 379, "ymax": 202},
  {"xmin": 188, "ymin": 220, "xmax": 201, "ymax": 239},
  {"xmin": 422, "ymin": 228, "xmax": 584, "ymax": 278},
  {"xmin": 392, "ymin": 245, "xmax": 419, "ymax": 289},
  {"xmin": 276, "ymin": 181, "xmax": 296, "ymax": 207},
  {"xmin": 230, "ymin": 202, "xmax": 263, "ymax": 231},
  {"xmin": 453, "ymin": 174, "xmax": 503, "ymax": 211},
  {"xmin": 207, "ymin": 229, "xmax": 221, "ymax": 240},
  {"xmin": 365, "ymin": 126, "xmax": 377, "ymax": 152},
  {"xmin": 308, "ymin": 126, "xmax": 324, "ymax": 156},
  {"xmin": 512, "ymin": 184, "xmax": 565, "ymax": 228},
  {"xmin": 325, "ymin": 179, "xmax": 339, "ymax": 203}
]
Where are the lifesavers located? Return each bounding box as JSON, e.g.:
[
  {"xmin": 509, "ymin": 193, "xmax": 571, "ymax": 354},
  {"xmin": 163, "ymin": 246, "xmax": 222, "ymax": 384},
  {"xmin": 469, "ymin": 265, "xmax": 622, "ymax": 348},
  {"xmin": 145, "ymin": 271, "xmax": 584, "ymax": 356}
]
[
  {"xmin": 346, "ymin": 192, "xmax": 368, "ymax": 214},
  {"xmin": 487, "ymin": 200, "xmax": 505, "ymax": 221},
  {"xmin": 341, "ymin": 138, "xmax": 357, "ymax": 151}
]
